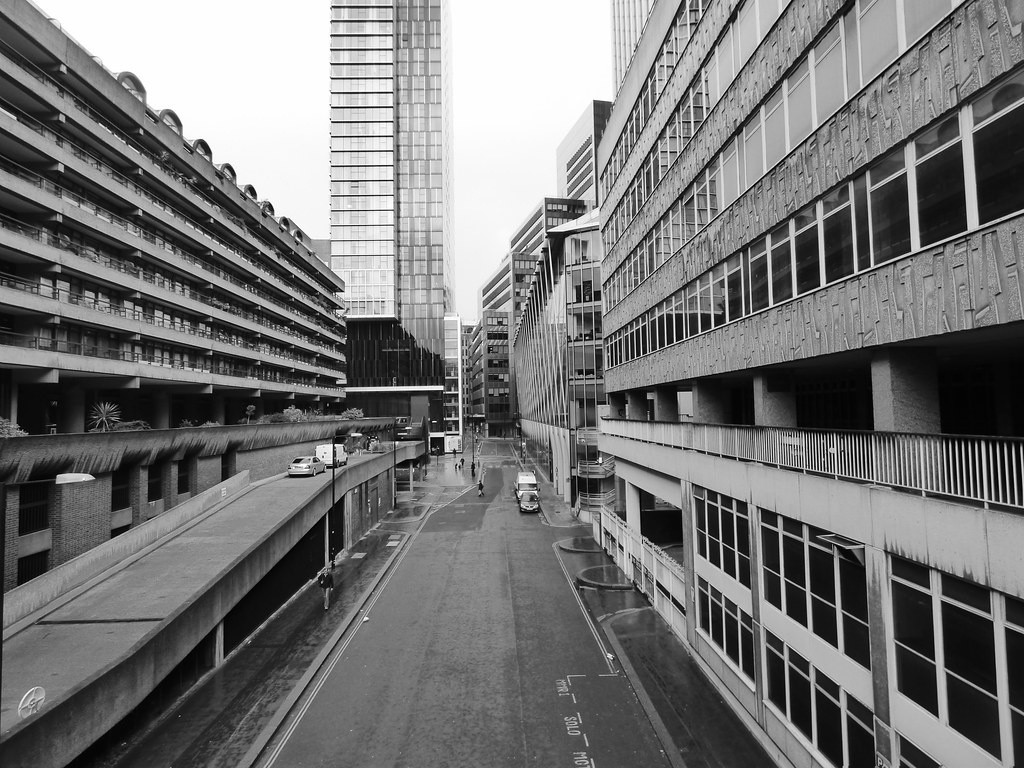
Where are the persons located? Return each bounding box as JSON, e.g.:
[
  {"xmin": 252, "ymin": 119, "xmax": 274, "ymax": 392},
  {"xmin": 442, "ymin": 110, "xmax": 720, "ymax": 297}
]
[
  {"xmin": 453, "ymin": 448, "xmax": 456, "ymax": 457},
  {"xmin": 318, "ymin": 568, "xmax": 334, "ymax": 609},
  {"xmin": 503, "ymin": 432, "xmax": 506, "ymax": 440},
  {"xmin": 471, "ymin": 462, "xmax": 476, "ymax": 477},
  {"xmin": 432, "ymin": 445, "xmax": 440, "ymax": 456},
  {"xmin": 454, "ymin": 457, "xmax": 465, "ymax": 470},
  {"xmin": 475, "ymin": 435, "xmax": 478, "ymax": 443},
  {"xmin": 478, "ymin": 480, "xmax": 484, "ymax": 497}
]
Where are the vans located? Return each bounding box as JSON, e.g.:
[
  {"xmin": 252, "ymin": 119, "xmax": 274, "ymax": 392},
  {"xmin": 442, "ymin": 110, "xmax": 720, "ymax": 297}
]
[
  {"xmin": 314, "ymin": 443, "xmax": 349, "ymax": 467},
  {"xmin": 513, "ymin": 470, "xmax": 541, "ymax": 503}
]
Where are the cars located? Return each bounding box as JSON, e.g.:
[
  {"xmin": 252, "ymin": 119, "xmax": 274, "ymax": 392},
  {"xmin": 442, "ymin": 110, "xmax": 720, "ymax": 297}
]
[
  {"xmin": 519, "ymin": 492, "xmax": 540, "ymax": 513},
  {"xmin": 287, "ymin": 456, "xmax": 326, "ymax": 478}
]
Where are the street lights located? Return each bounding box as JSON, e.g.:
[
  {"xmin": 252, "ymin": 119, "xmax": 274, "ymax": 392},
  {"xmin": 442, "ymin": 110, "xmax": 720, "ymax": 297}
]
[
  {"xmin": 424, "ymin": 420, "xmax": 438, "ymax": 439},
  {"xmin": 392, "ymin": 427, "xmax": 412, "ymax": 449},
  {"xmin": 332, "ymin": 432, "xmax": 362, "ymax": 491}
]
